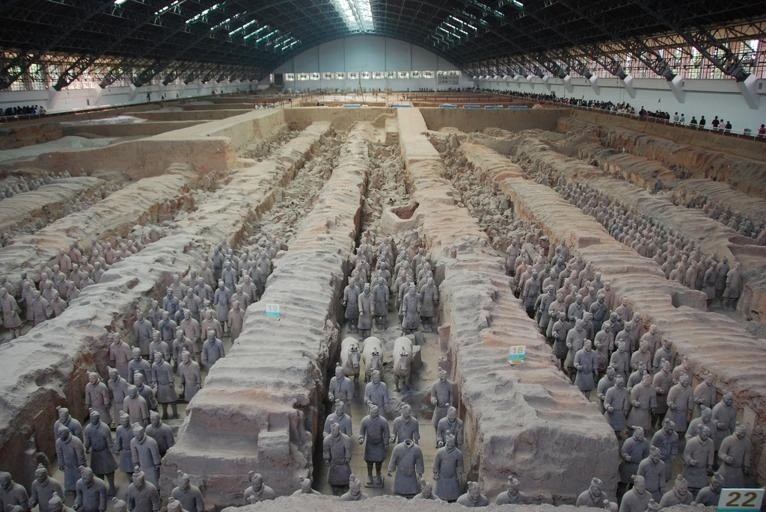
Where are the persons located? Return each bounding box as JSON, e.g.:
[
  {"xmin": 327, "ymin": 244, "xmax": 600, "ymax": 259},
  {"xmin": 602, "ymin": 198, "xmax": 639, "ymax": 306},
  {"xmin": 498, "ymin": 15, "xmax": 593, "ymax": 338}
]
[
  {"xmin": 212, "ymin": 88, "xmax": 240, "ymax": 94},
  {"xmin": 718, "ymin": 119, "xmax": 726, "ymax": 133},
  {"xmin": 725, "ymin": 120, "xmax": 732, "ymax": 133},
  {"xmin": 712, "ymin": 116, "xmax": 719, "ymax": 131},
  {"xmin": 408, "ymin": 86, "xmax": 475, "ymax": 92},
  {"xmin": 673, "ymin": 112, "xmax": 679, "ymax": 126},
  {"xmin": 476, "ymin": 86, "xmax": 556, "ymax": 102},
  {"xmin": 699, "ymin": 115, "xmax": 706, "ymax": 130},
  {"xmin": 680, "ymin": 113, "xmax": 685, "ymax": 126},
  {"xmin": 690, "ymin": 116, "xmax": 697, "ymax": 128},
  {"xmin": 1, "ymin": 105, "xmax": 48, "ymax": 123},
  {"xmin": 176, "ymin": 93, "xmax": 179, "ymax": 97},
  {"xmin": 757, "ymin": 124, "xmax": 766, "ymax": 140},
  {"xmin": 1, "ymin": 128, "xmax": 766, "ymax": 511},
  {"xmin": 161, "ymin": 95, "xmax": 164, "ymax": 99},
  {"xmin": 558, "ymin": 96, "xmax": 635, "ymax": 114},
  {"xmin": 146, "ymin": 93, "xmax": 150, "ymax": 104},
  {"xmin": 639, "ymin": 106, "xmax": 670, "ymax": 119}
]
